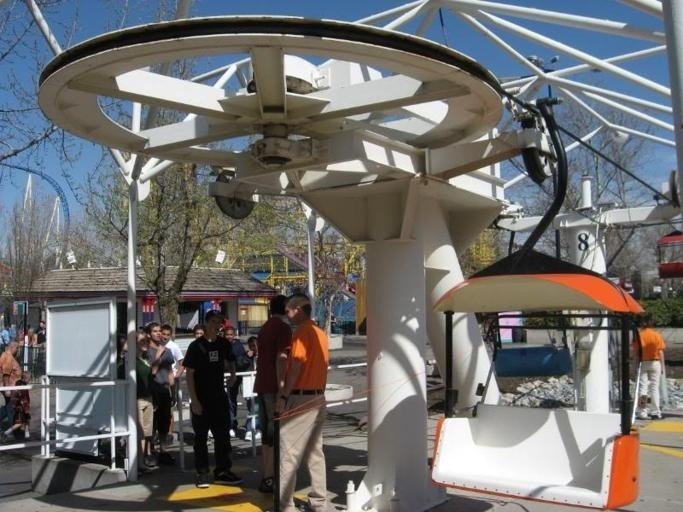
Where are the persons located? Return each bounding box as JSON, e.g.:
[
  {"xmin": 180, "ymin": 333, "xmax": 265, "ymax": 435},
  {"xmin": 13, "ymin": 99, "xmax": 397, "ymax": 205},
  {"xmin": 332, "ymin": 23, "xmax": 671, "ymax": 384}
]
[
  {"xmin": 631, "ymin": 320, "xmax": 667, "ymax": 421},
  {"xmin": 1, "ymin": 312, "xmax": 47, "ymax": 446},
  {"xmin": 182, "ymin": 308, "xmax": 244, "ymax": 492},
  {"xmin": 329, "ymin": 311, "xmax": 337, "ymax": 334},
  {"xmin": 137, "ymin": 320, "xmax": 261, "ymax": 470},
  {"xmin": 254, "ymin": 294, "xmax": 293, "ymax": 494},
  {"xmin": 273, "ymin": 293, "xmax": 330, "ymax": 511}
]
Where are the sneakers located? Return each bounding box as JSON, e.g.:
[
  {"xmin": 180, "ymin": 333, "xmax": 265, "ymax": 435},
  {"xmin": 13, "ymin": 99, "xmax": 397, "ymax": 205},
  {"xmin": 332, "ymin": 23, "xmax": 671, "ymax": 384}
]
[
  {"xmin": 214, "ymin": 470, "xmax": 242, "ymax": 484},
  {"xmin": 195, "ymin": 472, "xmax": 209, "ymax": 487},
  {"xmin": 260, "ymin": 477, "xmax": 273, "ymax": 492}
]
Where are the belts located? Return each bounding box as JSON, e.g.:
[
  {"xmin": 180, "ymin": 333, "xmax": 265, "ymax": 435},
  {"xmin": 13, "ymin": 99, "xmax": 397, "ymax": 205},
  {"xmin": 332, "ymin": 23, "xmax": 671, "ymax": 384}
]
[{"xmin": 290, "ymin": 389, "xmax": 323, "ymax": 394}]
